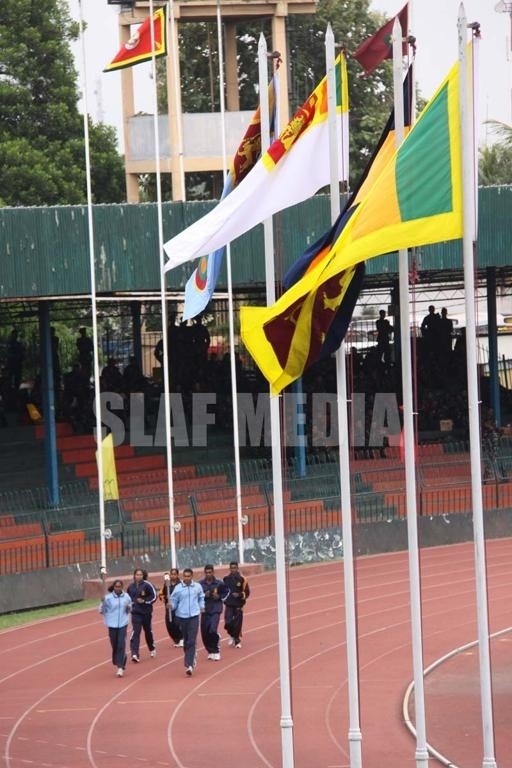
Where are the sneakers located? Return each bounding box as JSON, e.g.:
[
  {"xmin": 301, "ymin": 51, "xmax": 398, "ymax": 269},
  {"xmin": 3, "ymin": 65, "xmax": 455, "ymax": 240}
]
[
  {"xmin": 131, "ymin": 654, "xmax": 140, "ymax": 663},
  {"xmin": 173, "ymin": 639, "xmax": 183, "ymax": 647},
  {"xmin": 207, "ymin": 652, "xmax": 220, "ymax": 661},
  {"xmin": 116, "ymin": 667, "xmax": 124, "ymax": 677},
  {"xmin": 228, "ymin": 637, "xmax": 235, "ymax": 647},
  {"xmin": 150, "ymin": 644, "xmax": 156, "ymax": 656},
  {"xmin": 185, "ymin": 665, "xmax": 192, "ymax": 675},
  {"xmin": 235, "ymin": 642, "xmax": 241, "ymax": 648}
]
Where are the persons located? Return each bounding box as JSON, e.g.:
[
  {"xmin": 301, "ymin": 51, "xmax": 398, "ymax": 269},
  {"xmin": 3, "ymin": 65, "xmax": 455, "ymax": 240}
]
[
  {"xmin": 199, "ymin": 565, "xmax": 231, "ymax": 661},
  {"xmin": 102, "ymin": 357, "xmax": 149, "ymax": 432},
  {"xmin": 2, "ymin": 327, "xmax": 97, "ymax": 433},
  {"xmin": 349, "ymin": 311, "xmax": 394, "ymax": 392},
  {"xmin": 422, "ymin": 392, "xmax": 511, "ymax": 450},
  {"xmin": 98, "ymin": 579, "xmax": 133, "ymax": 677},
  {"xmin": 223, "ymin": 562, "xmax": 251, "ymax": 647},
  {"xmin": 159, "ymin": 569, "xmax": 184, "ymax": 647},
  {"xmin": 287, "ymin": 366, "xmax": 335, "ymax": 393},
  {"xmin": 127, "ymin": 569, "xmax": 157, "ymax": 661},
  {"xmin": 165, "ymin": 569, "xmax": 205, "ymax": 676},
  {"xmin": 153, "ymin": 317, "xmax": 232, "ymax": 429},
  {"xmin": 421, "ymin": 306, "xmax": 468, "ymax": 400}
]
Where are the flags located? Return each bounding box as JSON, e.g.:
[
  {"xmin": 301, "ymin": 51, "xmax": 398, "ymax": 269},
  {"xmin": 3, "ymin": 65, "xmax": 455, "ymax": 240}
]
[
  {"xmin": 353, "ymin": 6, "xmax": 406, "ymax": 75},
  {"xmin": 103, "ymin": 6, "xmax": 167, "ymax": 75},
  {"xmin": 239, "ymin": 40, "xmax": 475, "ymax": 400},
  {"xmin": 179, "ymin": 78, "xmax": 274, "ymax": 321},
  {"xmin": 285, "ymin": 71, "xmax": 410, "ymax": 377},
  {"xmin": 159, "ymin": 48, "xmax": 350, "ymax": 273}
]
